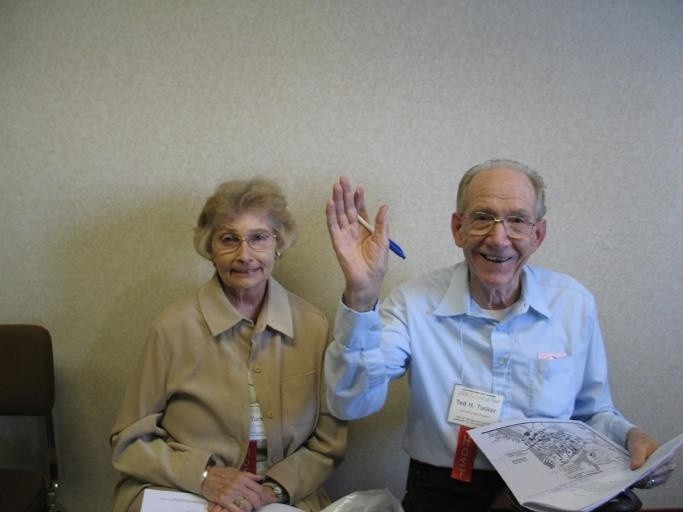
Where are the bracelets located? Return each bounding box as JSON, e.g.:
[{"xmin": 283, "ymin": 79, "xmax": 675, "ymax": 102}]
[
  {"xmin": 200, "ymin": 462, "xmax": 214, "ymax": 486},
  {"xmin": 262, "ymin": 481, "xmax": 285, "ymax": 503}
]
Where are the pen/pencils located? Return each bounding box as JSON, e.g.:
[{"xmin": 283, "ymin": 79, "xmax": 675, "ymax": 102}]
[{"xmin": 357, "ymin": 214, "xmax": 406, "ymax": 259}]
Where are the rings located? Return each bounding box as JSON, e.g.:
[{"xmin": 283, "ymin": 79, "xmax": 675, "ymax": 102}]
[
  {"xmin": 651, "ymin": 480, "xmax": 655, "ymax": 486},
  {"xmin": 233, "ymin": 498, "xmax": 246, "ymax": 508}
]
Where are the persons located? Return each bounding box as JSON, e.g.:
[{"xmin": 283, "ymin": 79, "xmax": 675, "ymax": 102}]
[
  {"xmin": 110, "ymin": 174, "xmax": 348, "ymax": 512},
  {"xmin": 323, "ymin": 159, "xmax": 674, "ymax": 512}
]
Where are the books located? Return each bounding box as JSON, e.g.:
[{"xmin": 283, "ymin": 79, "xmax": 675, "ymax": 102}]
[{"xmin": 139, "ymin": 487, "xmax": 308, "ymax": 512}]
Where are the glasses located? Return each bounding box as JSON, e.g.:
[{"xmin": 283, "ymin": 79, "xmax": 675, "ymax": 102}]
[
  {"xmin": 210, "ymin": 228, "xmax": 277, "ymax": 251},
  {"xmin": 461, "ymin": 209, "xmax": 538, "ymax": 241}
]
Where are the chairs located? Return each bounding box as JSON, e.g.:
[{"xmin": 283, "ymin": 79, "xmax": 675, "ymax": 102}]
[{"xmin": 1, "ymin": 323, "xmax": 62, "ymax": 511}]
